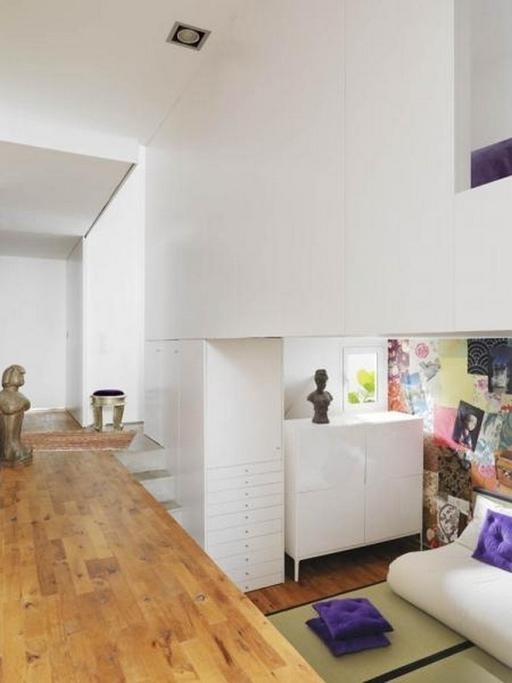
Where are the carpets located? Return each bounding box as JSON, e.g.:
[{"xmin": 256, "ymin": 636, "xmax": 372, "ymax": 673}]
[
  {"xmin": 264, "ymin": 579, "xmax": 512, "ymax": 682},
  {"xmin": 19, "ymin": 431, "xmax": 137, "ymax": 451}
]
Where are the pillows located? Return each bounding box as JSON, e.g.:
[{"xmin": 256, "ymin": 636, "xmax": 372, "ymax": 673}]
[
  {"xmin": 470, "ymin": 509, "xmax": 512, "ymax": 574},
  {"xmin": 305, "ymin": 598, "xmax": 395, "ymax": 657}
]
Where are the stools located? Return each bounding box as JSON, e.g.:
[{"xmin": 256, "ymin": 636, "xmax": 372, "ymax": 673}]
[{"xmin": 90, "ymin": 390, "xmax": 127, "ymax": 432}]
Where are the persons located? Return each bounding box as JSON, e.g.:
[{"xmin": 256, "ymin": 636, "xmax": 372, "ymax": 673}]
[
  {"xmin": 308, "ymin": 368, "xmax": 335, "ymax": 424},
  {"xmin": 0, "ymin": 363, "xmax": 34, "ymax": 469},
  {"xmin": 453, "ymin": 412, "xmax": 479, "ymax": 450}
]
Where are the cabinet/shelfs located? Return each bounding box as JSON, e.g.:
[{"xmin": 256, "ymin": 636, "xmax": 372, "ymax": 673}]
[
  {"xmin": 284, "ymin": 410, "xmax": 424, "ymax": 582},
  {"xmin": 180, "ymin": 337, "xmax": 285, "ymax": 593}
]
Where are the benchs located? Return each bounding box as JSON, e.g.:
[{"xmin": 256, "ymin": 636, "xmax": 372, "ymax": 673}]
[{"xmin": 386, "ymin": 486, "xmax": 512, "ymax": 670}]
